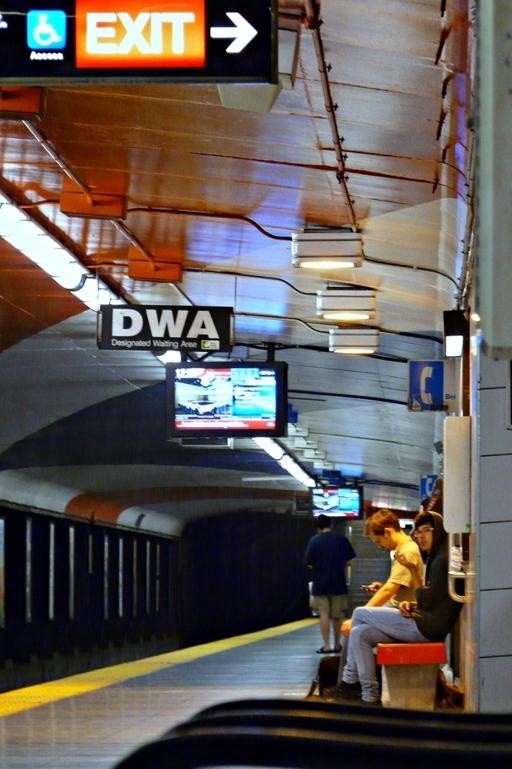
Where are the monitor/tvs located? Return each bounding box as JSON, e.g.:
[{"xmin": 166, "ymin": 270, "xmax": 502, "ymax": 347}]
[
  {"xmin": 309, "ymin": 486, "xmax": 363, "ymax": 521},
  {"xmin": 164, "ymin": 362, "xmax": 288, "ymax": 437}
]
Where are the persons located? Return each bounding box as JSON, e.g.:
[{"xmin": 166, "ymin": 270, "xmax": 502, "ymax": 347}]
[
  {"xmin": 323, "ymin": 510, "xmax": 466, "ymax": 709},
  {"xmin": 334, "ymin": 506, "xmax": 428, "ymax": 701},
  {"xmin": 303, "ymin": 514, "xmax": 356, "ymax": 654}
]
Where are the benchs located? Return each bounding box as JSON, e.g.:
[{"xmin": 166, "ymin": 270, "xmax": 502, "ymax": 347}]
[{"xmin": 374, "ymin": 642, "xmax": 447, "ymax": 712}]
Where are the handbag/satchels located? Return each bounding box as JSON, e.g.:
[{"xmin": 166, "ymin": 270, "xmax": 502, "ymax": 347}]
[{"xmin": 317, "ymin": 656, "xmax": 341, "ymax": 696}]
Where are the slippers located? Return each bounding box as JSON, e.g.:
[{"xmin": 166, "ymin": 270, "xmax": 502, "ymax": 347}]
[{"xmin": 316, "ymin": 643, "xmax": 342, "ymax": 653}]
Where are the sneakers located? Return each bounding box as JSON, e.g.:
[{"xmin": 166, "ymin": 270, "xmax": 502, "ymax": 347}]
[{"xmin": 324, "ymin": 681, "xmax": 362, "ymax": 699}]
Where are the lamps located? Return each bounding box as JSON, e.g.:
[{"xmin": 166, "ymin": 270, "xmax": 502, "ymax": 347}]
[
  {"xmin": 444, "ymin": 310, "xmax": 465, "ymax": 358},
  {"xmin": 329, "ymin": 328, "xmax": 380, "ymax": 355},
  {"xmin": 290, "ymin": 226, "xmax": 363, "ymax": 269},
  {"xmin": 317, "ymin": 286, "xmax": 376, "ymax": 322}
]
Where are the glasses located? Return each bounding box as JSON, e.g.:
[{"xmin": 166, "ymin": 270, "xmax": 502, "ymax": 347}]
[{"xmin": 412, "ymin": 527, "xmax": 434, "ymax": 538}]
